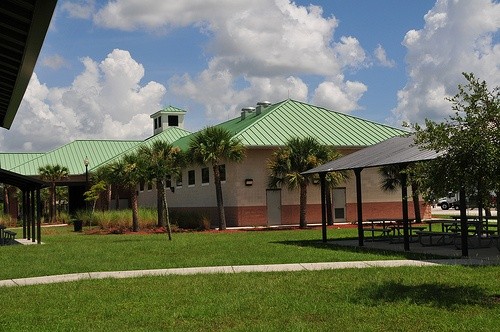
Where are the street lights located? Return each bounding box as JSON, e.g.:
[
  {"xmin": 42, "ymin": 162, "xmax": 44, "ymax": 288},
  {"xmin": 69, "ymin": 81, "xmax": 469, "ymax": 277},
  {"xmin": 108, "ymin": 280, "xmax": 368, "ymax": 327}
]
[{"xmin": 84, "ymin": 156, "xmax": 90, "ymax": 214}]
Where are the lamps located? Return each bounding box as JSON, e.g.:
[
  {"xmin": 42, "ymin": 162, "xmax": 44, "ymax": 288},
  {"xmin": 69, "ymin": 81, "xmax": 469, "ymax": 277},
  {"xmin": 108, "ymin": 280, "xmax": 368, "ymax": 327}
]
[
  {"xmin": 313, "ymin": 177, "xmax": 320, "ymax": 184},
  {"xmin": 245, "ymin": 179, "xmax": 253, "ymax": 184}
]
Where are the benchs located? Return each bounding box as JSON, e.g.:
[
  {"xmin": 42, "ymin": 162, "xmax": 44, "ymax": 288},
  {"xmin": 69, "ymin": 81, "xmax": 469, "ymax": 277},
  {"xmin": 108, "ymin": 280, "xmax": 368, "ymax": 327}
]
[
  {"xmin": 4, "ymin": 231, "xmax": 17, "ymax": 245},
  {"xmin": 361, "ymin": 224, "xmax": 500, "ymax": 238}
]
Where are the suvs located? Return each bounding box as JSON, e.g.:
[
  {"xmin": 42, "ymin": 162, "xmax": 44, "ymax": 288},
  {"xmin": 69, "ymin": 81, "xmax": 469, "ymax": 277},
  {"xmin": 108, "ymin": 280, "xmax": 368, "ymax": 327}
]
[{"xmin": 437, "ymin": 189, "xmax": 497, "ymax": 210}]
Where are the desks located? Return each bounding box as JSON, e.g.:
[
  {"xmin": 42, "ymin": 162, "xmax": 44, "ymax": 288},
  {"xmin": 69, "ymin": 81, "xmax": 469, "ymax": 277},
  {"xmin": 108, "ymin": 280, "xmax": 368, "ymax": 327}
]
[
  {"xmin": 421, "ymin": 217, "xmax": 486, "ymax": 247},
  {"xmin": 0, "ymin": 225, "xmax": 7, "ymax": 246},
  {"xmin": 366, "ymin": 218, "xmax": 419, "ymax": 244}
]
